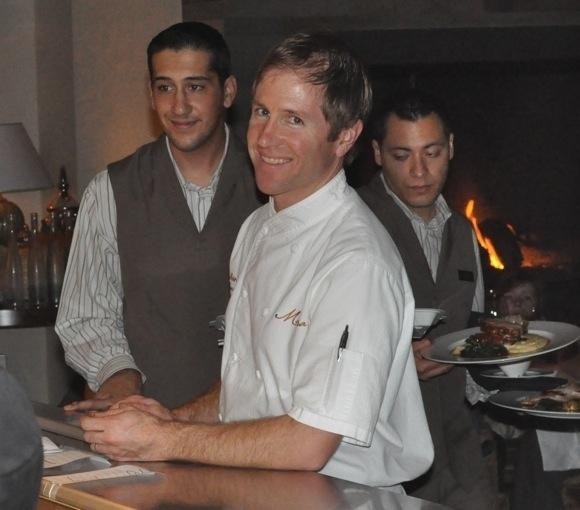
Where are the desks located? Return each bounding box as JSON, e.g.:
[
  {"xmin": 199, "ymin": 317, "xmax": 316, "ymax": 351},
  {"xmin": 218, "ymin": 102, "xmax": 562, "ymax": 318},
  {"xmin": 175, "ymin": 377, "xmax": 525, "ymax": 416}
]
[
  {"xmin": 29, "ymin": 399, "xmax": 449, "ymax": 510},
  {"xmin": 1, "ymin": 315, "xmax": 79, "ymax": 409}
]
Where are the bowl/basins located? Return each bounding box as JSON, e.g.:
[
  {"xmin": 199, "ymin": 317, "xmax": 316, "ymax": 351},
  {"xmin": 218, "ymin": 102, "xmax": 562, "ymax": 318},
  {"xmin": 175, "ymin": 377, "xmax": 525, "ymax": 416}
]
[
  {"xmin": 411, "ymin": 308, "xmax": 442, "ymax": 339},
  {"xmin": 498, "ymin": 359, "xmax": 531, "ymax": 376}
]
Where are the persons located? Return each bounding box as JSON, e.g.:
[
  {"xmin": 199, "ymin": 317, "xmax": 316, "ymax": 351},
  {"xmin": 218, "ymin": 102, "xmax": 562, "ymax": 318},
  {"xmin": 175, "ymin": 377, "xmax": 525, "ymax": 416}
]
[
  {"xmin": 54, "ymin": 20, "xmax": 264, "ymax": 411},
  {"xmin": 347, "ymin": 87, "xmax": 515, "ymax": 510},
  {"xmin": 60, "ymin": 28, "xmax": 435, "ymax": 497},
  {"xmin": 495, "ymin": 270, "xmax": 542, "ymax": 321}
]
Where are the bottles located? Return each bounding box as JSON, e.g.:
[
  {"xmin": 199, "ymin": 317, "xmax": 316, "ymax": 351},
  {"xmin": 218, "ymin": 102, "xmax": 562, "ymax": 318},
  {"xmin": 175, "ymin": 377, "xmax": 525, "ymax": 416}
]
[{"xmin": 0, "ymin": 166, "xmax": 80, "ymax": 310}]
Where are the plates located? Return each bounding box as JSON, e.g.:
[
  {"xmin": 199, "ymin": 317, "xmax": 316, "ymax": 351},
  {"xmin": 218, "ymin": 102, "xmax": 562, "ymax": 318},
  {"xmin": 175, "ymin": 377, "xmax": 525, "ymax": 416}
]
[
  {"xmin": 419, "ymin": 319, "xmax": 580, "ymax": 365},
  {"xmin": 488, "ymin": 389, "xmax": 579, "ymax": 421},
  {"xmin": 479, "ymin": 367, "xmax": 560, "ymax": 378}
]
